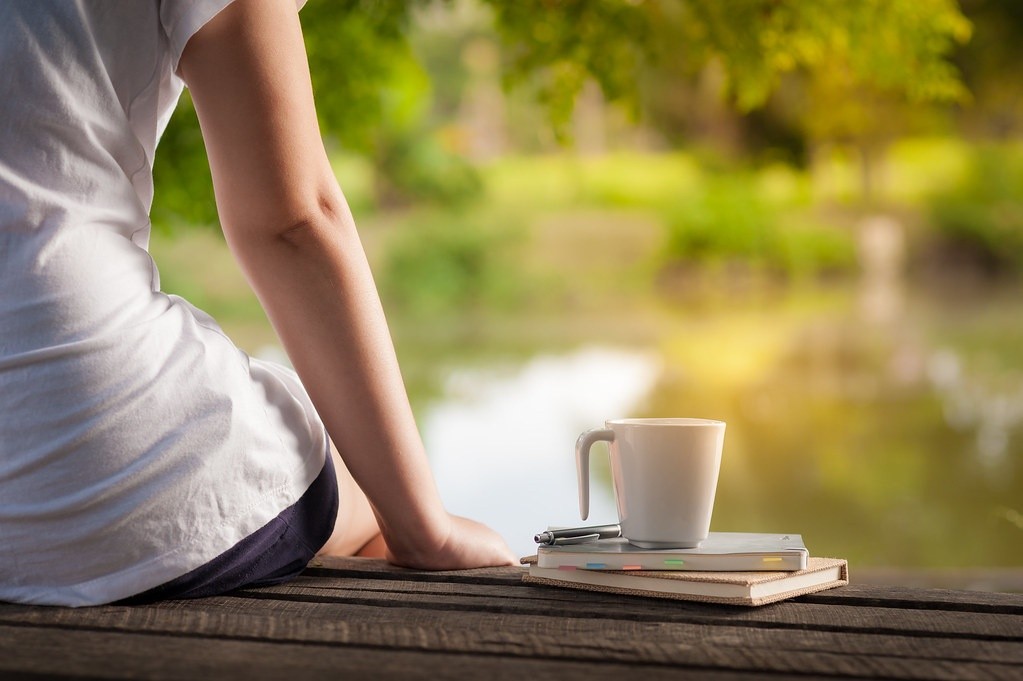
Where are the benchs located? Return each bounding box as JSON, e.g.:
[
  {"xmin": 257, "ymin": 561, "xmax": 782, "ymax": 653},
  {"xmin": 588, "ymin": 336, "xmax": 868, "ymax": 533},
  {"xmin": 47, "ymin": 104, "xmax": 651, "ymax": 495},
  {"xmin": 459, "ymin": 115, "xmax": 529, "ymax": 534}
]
[{"xmin": 0, "ymin": 556, "xmax": 1023, "ymax": 681}]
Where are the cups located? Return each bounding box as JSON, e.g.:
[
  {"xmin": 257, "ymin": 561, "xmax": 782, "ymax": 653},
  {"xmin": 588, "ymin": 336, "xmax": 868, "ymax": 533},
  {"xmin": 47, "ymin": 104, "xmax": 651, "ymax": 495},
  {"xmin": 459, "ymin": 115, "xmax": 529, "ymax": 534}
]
[{"xmin": 575, "ymin": 418, "xmax": 727, "ymax": 548}]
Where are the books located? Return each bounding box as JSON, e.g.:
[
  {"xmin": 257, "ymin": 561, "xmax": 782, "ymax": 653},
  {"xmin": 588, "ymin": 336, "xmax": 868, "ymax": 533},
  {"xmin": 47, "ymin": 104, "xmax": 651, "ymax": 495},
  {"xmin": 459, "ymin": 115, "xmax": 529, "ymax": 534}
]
[{"xmin": 528, "ymin": 528, "xmax": 851, "ymax": 607}]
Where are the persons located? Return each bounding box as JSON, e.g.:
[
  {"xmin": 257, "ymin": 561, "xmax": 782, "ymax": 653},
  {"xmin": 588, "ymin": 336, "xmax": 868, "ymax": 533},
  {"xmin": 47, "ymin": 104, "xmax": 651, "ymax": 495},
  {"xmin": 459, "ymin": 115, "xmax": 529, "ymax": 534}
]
[{"xmin": 1, "ymin": 0, "xmax": 522, "ymax": 606}]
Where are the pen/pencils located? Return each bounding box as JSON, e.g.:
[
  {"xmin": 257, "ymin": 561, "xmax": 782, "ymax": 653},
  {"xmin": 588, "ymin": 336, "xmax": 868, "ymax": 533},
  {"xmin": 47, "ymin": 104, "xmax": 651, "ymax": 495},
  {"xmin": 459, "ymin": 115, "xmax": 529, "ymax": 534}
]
[{"xmin": 535, "ymin": 523, "xmax": 622, "ymax": 546}]
